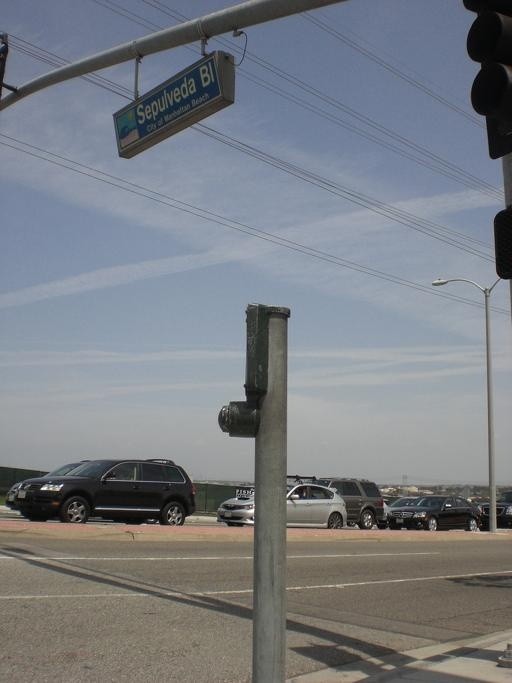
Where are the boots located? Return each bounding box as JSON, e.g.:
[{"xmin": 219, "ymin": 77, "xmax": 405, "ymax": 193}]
[
  {"xmin": 310, "ymin": 478, "xmax": 384, "ymax": 530},
  {"xmin": 5, "ymin": 459, "xmax": 91, "ymax": 518},
  {"xmin": 12, "ymin": 458, "xmax": 197, "ymax": 526}
]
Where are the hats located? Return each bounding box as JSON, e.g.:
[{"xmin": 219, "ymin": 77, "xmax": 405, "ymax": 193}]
[
  {"xmin": 216, "ymin": 482, "xmax": 349, "ymax": 530},
  {"xmin": 377, "ymin": 490, "xmax": 512, "ymax": 533}
]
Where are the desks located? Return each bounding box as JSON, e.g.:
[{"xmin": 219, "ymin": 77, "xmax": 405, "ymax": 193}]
[{"xmin": 431, "ymin": 277, "xmax": 498, "ymax": 534}]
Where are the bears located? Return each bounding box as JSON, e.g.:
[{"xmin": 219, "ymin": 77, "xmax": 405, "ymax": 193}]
[{"xmin": 217, "ymin": 302, "xmax": 289, "ymax": 682}]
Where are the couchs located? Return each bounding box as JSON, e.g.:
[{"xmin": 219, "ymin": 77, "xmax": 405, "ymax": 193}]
[{"xmin": 460, "ymin": 2, "xmax": 510, "ymax": 159}]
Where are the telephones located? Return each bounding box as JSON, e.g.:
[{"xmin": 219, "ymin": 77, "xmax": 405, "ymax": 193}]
[{"xmin": 111, "ymin": 49, "xmax": 235, "ymax": 160}]
